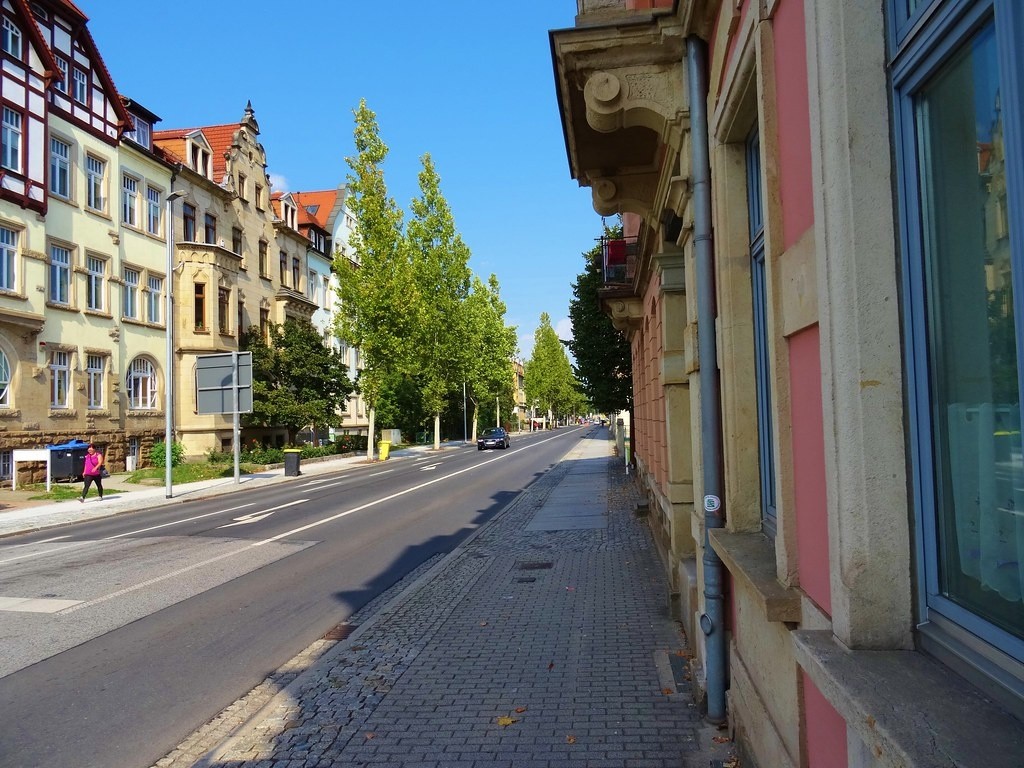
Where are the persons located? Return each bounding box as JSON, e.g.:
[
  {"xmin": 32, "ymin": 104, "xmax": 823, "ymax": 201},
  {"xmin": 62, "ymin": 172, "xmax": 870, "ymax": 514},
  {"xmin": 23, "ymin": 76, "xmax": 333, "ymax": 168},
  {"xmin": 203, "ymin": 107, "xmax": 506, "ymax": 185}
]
[{"xmin": 76, "ymin": 444, "xmax": 104, "ymax": 503}]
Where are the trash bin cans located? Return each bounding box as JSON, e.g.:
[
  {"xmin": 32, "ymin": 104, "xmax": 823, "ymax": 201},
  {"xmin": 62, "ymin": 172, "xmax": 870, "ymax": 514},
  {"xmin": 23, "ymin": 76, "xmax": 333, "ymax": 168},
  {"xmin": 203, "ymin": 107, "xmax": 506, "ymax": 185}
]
[
  {"xmin": 46, "ymin": 439, "xmax": 90, "ymax": 482},
  {"xmin": 282, "ymin": 447, "xmax": 304, "ymax": 477},
  {"xmin": 377, "ymin": 440, "xmax": 393, "ymax": 462}
]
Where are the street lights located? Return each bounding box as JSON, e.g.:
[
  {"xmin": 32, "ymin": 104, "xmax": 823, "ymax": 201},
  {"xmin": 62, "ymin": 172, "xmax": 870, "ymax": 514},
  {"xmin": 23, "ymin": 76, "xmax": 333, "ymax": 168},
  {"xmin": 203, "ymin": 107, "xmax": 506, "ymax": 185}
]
[{"xmin": 164, "ymin": 191, "xmax": 186, "ymax": 496}]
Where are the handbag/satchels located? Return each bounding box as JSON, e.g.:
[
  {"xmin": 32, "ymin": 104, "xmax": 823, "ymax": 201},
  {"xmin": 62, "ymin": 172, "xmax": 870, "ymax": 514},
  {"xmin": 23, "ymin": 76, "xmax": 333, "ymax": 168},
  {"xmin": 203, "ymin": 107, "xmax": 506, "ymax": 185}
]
[{"xmin": 100, "ymin": 466, "xmax": 110, "ymax": 478}]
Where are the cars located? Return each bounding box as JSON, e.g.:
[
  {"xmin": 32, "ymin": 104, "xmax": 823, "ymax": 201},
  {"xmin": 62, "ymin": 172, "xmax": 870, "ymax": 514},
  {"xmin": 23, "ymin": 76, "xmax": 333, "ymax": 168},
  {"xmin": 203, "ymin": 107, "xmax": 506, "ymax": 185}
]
[{"xmin": 478, "ymin": 427, "xmax": 511, "ymax": 450}]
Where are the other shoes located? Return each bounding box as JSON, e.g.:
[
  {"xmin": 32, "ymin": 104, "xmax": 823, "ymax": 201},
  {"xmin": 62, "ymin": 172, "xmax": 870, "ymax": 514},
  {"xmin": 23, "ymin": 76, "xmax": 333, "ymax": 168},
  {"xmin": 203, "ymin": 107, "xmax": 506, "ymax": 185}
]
[
  {"xmin": 98, "ymin": 497, "xmax": 104, "ymax": 501},
  {"xmin": 77, "ymin": 497, "xmax": 84, "ymax": 503}
]
